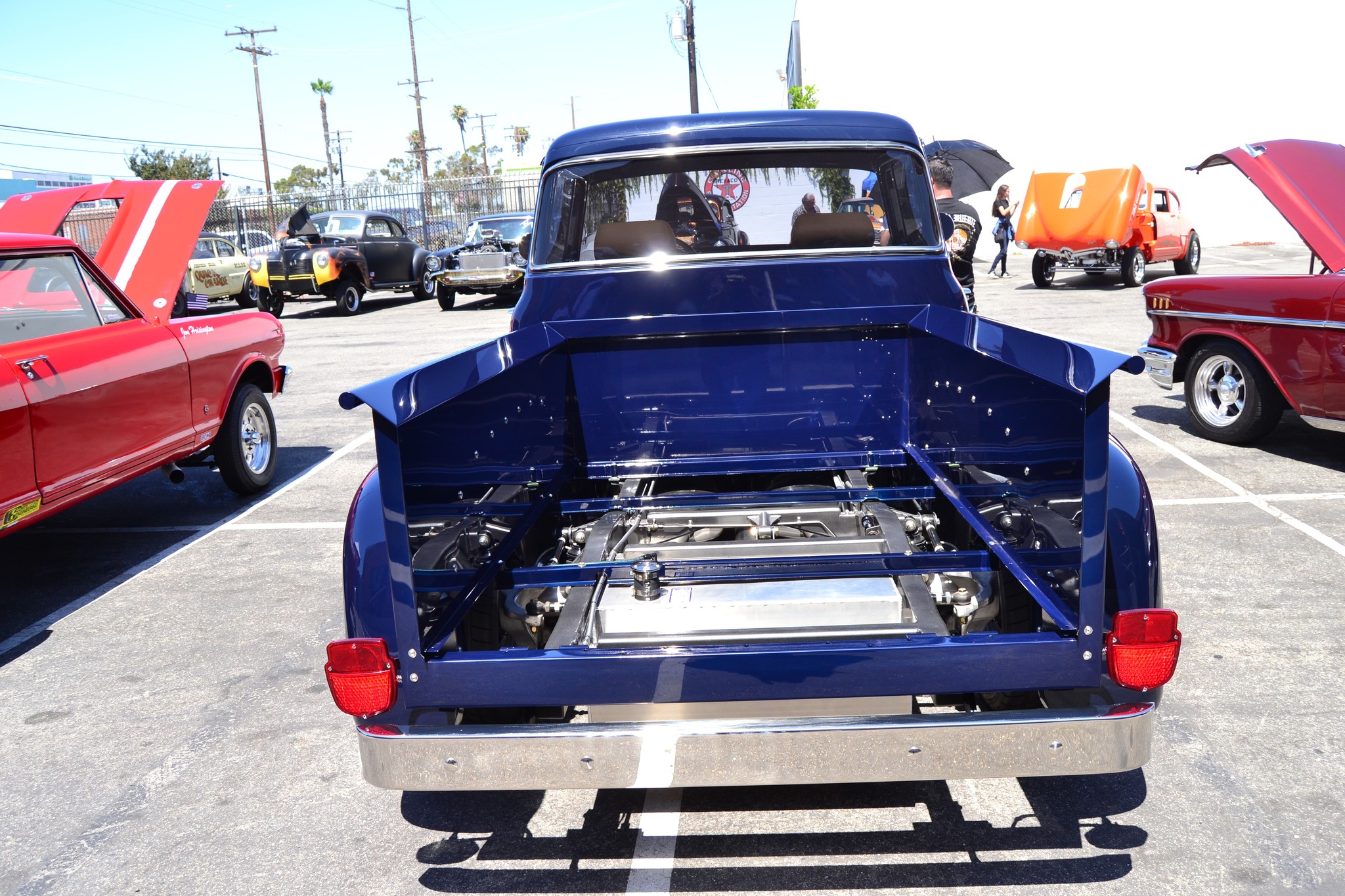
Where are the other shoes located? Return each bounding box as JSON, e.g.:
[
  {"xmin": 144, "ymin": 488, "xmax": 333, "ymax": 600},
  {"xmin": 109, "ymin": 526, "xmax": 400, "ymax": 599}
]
[
  {"xmin": 1001, "ymin": 272, "xmax": 1012, "ymax": 278},
  {"xmin": 987, "ymin": 271, "xmax": 999, "ymax": 278}
]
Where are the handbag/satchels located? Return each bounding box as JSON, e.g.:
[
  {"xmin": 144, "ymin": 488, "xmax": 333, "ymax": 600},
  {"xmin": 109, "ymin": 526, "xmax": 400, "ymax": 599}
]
[{"xmin": 994, "ymin": 231, "xmax": 1004, "ymax": 243}]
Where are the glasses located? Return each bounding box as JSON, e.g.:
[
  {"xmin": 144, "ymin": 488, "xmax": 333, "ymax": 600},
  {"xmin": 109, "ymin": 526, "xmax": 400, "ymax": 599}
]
[{"xmin": 1005, "ymin": 190, "xmax": 1010, "ymax": 193}]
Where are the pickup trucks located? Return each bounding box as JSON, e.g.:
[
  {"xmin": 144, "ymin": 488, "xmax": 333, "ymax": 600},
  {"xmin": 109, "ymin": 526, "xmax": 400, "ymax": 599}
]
[{"xmin": 319, "ymin": 107, "xmax": 1183, "ymax": 794}]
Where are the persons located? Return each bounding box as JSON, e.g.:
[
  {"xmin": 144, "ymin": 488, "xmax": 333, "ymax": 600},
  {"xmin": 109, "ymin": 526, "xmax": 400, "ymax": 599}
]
[
  {"xmin": 886, "ymin": 156, "xmax": 982, "ymax": 314},
  {"xmin": 987, "ymin": 184, "xmax": 1020, "ymax": 279},
  {"xmin": 790, "ymin": 193, "xmax": 821, "ymax": 227}
]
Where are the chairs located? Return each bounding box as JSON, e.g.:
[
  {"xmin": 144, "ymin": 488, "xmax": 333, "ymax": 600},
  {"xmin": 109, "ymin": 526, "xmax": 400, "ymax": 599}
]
[
  {"xmin": 790, "ymin": 213, "xmax": 875, "ymax": 248},
  {"xmin": 595, "ymin": 221, "xmax": 678, "ymax": 261}
]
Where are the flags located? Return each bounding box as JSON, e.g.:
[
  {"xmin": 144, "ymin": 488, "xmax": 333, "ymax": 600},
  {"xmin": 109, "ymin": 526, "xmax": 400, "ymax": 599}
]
[{"xmin": 186, "ymin": 293, "xmax": 208, "ymax": 311}]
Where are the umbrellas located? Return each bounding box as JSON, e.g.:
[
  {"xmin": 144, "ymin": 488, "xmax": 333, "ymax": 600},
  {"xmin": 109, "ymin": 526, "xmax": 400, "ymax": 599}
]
[{"xmin": 860, "ymin": 136, "xmax": 1014, "ymax": 200}]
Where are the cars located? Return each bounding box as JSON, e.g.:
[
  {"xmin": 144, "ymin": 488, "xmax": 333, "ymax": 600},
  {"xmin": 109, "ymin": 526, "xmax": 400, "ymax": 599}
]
[
  {"xmin": 1014, "ymin": 163, "xmax": 1201, "ymax": 288},
  {"xmin": 0, "ymin": 181, "xmax": 281, "ymax": 536},
  {"xmin": 1138, "ymin": 137, "xmax": 1345, "ymax": 446},
  {"xmin": 177, "ymin": 208, "xmax": 540, "ymax": 321}
]
